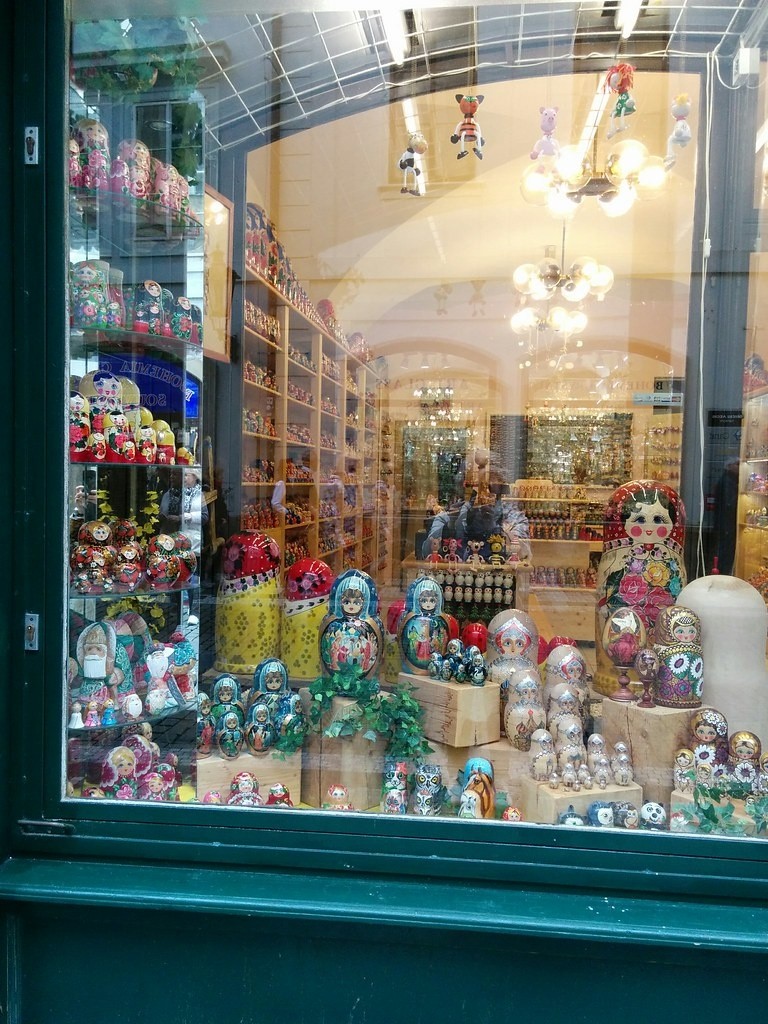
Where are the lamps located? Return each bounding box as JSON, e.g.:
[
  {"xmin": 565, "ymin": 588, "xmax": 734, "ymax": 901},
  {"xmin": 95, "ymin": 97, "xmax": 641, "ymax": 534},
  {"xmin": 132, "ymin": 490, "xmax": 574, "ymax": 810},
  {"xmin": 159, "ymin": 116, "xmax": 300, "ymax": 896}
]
[
  {"xmin": 512, "ymin": 219, "xmax": 616, "ymax": 300},
  {"xmin": 510, "ymin": 302, "xmax": 595, "ymax": 337},
  {"xmin": 523, "ymin": 71, "xmax": 669, "ymax": 216}
]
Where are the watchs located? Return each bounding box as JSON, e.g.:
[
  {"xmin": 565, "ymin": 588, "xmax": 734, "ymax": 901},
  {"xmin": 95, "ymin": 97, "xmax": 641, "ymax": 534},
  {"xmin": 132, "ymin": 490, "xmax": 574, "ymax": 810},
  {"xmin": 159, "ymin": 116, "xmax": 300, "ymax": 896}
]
[{"xmin": 73, "ymin": 508, "xmax": 84, "ymax": 518}]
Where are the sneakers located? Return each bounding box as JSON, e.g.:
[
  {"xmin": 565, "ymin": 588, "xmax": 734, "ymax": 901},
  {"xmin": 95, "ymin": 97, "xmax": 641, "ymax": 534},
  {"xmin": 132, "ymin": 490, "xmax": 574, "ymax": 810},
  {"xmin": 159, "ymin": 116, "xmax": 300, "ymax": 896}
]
[{"xmin": 187, "ymin": 614, "xmax": 199, "ymax": 624}]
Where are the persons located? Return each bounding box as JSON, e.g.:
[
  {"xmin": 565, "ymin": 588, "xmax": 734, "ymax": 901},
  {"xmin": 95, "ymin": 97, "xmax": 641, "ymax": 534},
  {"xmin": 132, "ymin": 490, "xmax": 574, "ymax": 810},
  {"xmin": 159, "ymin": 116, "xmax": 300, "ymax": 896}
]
[
  {"xmin": 270, "ymin": 450, "xmax": 315, "ymax": 514},
  {"xmin": 417, "ymin": 472, "xmax": 531, "ymax": 564},
  {"xmin": 68, "ymin": 463, "xmax": 208, "ymax": 625}
]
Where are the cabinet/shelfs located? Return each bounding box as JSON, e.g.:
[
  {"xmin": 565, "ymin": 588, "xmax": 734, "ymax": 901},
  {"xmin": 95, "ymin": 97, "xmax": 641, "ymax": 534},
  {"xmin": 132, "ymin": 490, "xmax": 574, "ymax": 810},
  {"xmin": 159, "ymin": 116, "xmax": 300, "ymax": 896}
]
[
  {"xmin": 735, "ymin": 385, "xmax": 768, "ymax": 604},
  {"xmin": 64, "ymin": 186, "xmax": 208, "ymax": 738},
  {"xmin": 241, "ymin": 261, "xmax": 393, "ymax": 589}
]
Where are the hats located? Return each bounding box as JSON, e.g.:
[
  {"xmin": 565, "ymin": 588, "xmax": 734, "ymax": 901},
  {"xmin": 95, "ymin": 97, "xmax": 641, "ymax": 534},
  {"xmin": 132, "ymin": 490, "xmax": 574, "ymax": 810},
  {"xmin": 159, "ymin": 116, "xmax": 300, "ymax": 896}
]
[{"xmin": 184, "ymin": 468, "xmax": 201, "ymax": 481}]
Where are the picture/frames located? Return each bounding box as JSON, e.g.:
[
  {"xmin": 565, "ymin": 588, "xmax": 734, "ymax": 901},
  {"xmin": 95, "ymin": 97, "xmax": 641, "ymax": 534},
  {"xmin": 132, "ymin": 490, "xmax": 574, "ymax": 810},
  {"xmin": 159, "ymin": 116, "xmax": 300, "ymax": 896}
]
[{"xmin": 203, "ymin": 187, "xmax": 235, "ymax": 368}]
